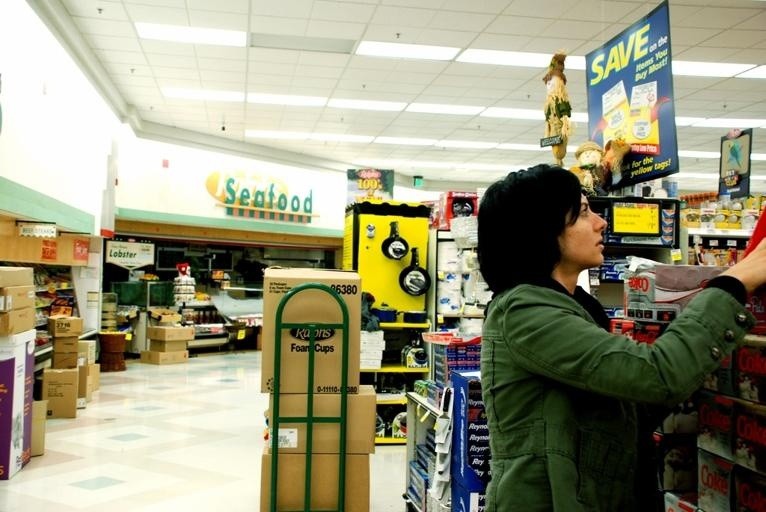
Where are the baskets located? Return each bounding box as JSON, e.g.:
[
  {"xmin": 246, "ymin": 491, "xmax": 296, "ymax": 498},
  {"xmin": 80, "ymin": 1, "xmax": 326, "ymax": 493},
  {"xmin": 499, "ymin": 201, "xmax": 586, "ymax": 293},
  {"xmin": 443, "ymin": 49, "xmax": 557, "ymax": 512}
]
[
  {"xmin": 97, "ymin": 352, "xmax": 127, "ymax": 372},
  {"xmin": 98, "ymin": 333, "xmax": 127, "ymax": 352}
]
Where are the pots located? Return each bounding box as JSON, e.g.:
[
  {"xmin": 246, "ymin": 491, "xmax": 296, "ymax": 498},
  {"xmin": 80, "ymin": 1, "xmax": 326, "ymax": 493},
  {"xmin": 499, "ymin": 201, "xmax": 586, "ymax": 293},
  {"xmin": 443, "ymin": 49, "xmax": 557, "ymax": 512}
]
[
  {"xmin": 400, "ymin": 310, "xmax": 428, "ymax": 323},
  {"xmin": 381, "ymin": 222, "xmax": 409, "ymax": 260},
  {"xmin": 371, "ymin": 303, "xmax": 399, "ymax": 323},
  {"xmin": 399, "ymin": 247, "xmax": 432, "ymax": 297}
]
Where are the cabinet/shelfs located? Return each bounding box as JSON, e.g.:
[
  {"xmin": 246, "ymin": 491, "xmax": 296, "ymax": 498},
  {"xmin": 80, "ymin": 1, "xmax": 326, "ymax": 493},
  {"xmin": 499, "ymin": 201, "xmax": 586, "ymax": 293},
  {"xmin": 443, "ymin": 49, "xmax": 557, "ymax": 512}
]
[
  {"xmin": 0, "ymin": 261, "xmax": 84, "ymax": 346},
  {"xmin": 665, "ymin": 333, "xmax": 766, "ymax": 511},
  {"xmin": 359, "ymin": 320, "xmax": 431, "ymax": 446},
  {"xmin": 452, "ymin": 371, "xmax": 491, "ymax": 512},
  {"xmin": 681, "ymin": 226, "xmax": 753, "ymax": 267},
  {"xmin": 406, "ymin": 392, "xmax": 452, "ymax": 512}
]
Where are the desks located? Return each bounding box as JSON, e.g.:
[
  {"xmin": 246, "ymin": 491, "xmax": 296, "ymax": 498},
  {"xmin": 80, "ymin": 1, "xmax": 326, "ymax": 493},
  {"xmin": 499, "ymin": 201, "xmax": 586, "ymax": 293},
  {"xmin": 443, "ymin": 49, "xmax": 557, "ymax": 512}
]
[{"xmin": 0, "ymin": 329, "xmax": 37, "ymax": 480}]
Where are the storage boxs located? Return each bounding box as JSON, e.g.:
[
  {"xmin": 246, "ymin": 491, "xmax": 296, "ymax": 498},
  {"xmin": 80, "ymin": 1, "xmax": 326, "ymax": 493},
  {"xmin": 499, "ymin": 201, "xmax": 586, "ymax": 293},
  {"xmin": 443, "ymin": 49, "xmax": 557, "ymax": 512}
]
[
  {"xmin": 140, "ymin": 350, "xmax": 189, "ymax": 365},
  {"xmin": 0, "ymin": 286, "xmax": 36, "ymax": 312},
  {"xmin": 48, "ymin": 317, "xmax": 83, "ymax": 337},
  {"xmin": 0, "ymin": 267, "xmax": 34, "ymax": 288},
  {"xmin": 30, "ymin": 336, "xmax": 100, "ymax": 455},
  {"xmin": 261, "ymin": 268, "xmax": 361, "ymax": 393},
  {"xmin": 146, "ymin": 327, "xmax": 195, "ymax": 341},
  {"xmin": 260, "ymin": 449, "xmax": 369, "ymax": 512},
  {"xmin": 150, "ymin": 340, "xmax": 186, "ymax": 352},
  {"xmin": 0, "ymin": 308, "xmax": 36, "ymax": 336},
  {"xmin": 270, "ymin": 385, "xmax": 376, "ymax": 454}
]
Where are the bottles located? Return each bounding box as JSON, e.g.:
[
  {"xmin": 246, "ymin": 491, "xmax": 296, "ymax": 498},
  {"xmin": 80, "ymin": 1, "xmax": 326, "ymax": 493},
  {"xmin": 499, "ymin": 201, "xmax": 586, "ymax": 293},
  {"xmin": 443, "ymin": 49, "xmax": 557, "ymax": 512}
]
[{"xmin": 680, "ymin": 193, "xmax": 718, "ymax": 209}]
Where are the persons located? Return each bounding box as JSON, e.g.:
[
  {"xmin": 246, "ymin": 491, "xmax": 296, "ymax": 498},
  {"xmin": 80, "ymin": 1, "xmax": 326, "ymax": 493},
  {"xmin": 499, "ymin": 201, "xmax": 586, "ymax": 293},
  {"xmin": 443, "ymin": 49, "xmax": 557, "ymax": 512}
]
[
  {"xmin": 541, "ymin": 52, "xmax": 571, "ymax": 166},
  {"xmin": 477, "ymin": 164, "xmax": 766, "ymax": 511},
  {"xmin": 569, "ymin": 139, "xmax": 632, "ymax": 197}
]
